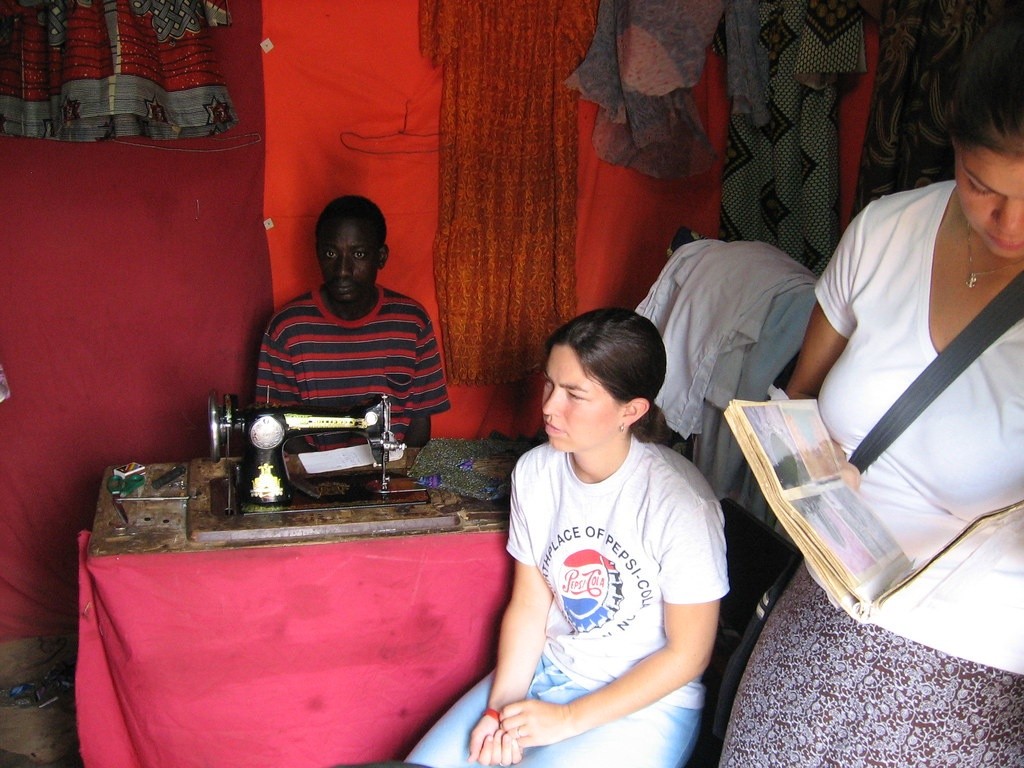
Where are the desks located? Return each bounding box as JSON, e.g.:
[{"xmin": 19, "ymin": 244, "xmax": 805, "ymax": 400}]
[{"xmin": 76, "ymin": 448, "xmax": 524, "ymax": 768}]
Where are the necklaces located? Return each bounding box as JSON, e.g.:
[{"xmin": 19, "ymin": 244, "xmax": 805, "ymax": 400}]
[{"xmin": 964, "ymin": 220, "xmax": 1024, "ymax": 288}]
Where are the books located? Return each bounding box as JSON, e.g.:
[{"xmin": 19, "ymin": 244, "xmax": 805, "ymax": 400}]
[{"xmin": 724, "ymin": 399, "xmax": 1024, "ymax": 676}]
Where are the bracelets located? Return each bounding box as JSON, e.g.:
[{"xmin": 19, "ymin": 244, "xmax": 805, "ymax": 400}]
[{"xmin": 482, "ymin": 707, "xmax": 499, "ymax": 720}]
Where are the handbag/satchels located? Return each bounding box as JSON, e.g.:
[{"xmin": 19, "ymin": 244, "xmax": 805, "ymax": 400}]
[{"xmin": 712, "ymin": 585, "xmax": 778, "ymax": 741}]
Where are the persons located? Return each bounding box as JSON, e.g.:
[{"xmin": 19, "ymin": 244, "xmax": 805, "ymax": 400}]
[
  {"xmin": 404, "ymin": 306, "xmax": 730, "ymax": 767},
  {"xmin": 255, "ymin": 195, "xmax": 451, "ymax": 447},
  {"xmin": 715, "ymin": 14, "xmax": 1024, "ymax": 767}
]
